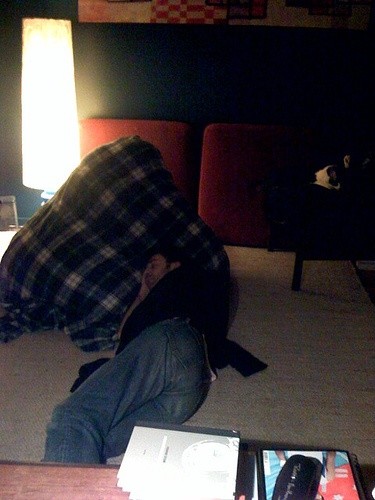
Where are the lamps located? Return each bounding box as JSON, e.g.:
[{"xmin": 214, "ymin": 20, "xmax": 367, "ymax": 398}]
[{"xmin": 21, "ymin": 18, "xmax": 81, "ymax": 207}]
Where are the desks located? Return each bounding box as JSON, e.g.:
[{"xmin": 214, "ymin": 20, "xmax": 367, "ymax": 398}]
[{"xmin": 268, "ymin": 209, "xmax": 375, "ymax": 291}]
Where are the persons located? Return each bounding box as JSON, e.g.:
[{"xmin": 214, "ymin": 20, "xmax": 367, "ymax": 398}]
[{"xmin": 42, "ymin": 246, "xmax": 211, "ymax": 464}]
